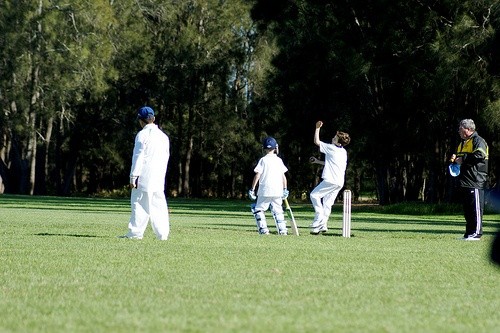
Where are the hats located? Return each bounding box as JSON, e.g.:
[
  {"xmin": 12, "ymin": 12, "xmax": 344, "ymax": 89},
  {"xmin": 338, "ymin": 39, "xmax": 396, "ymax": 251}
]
[
  {"xmin": 447, "ymin": 163, "xmax": 461, "ymax": 176},
  {"xmin": 262, "ymin": 136, "xmax": 278, "ymax": 149},
  {"xmin": 137, "ymin": 106, "xmax": 154, "ymax": 118}
]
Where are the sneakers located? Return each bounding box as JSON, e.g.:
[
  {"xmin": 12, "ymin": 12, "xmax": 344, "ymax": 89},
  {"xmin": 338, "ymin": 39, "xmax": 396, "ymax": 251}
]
[
  {"xmin": 310, "ymin": 226, "xmax": 328, "ymax": 234},
  {"xmin": 308, "ymin": 218, "xmax": 325, "ymax": 226}
]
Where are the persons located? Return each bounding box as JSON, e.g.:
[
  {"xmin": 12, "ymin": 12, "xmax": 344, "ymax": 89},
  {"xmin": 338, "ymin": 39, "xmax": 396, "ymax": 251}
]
[
  {"xmin": 308, "ymin": 121, "xmax": 351, "ymax": 235},
  {"xmin": 247, "ymin": 136, "xmax": 290, "ymax": 236},
  {"xmin": 448, "ymin": 118, "xmax": 489, "ymax": 242},
  {"xmin": 125, "ymin": 106, "xmax": 171, "ymax": 242}
]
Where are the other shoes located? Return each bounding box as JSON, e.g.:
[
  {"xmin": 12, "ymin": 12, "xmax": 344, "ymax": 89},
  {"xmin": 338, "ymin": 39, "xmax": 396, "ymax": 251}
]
[
  {"xmin": 460, "ymin": 235, "xmax": 483, "ymax": 240},
  {"xmin": 116, "ymin": 233, "xmax": 142, "ymax": 240}
]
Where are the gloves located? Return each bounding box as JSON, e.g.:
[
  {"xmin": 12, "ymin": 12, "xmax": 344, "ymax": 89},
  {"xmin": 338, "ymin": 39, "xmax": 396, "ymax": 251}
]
[
  {"xmin": 249, "ymin": 188, "xmax": 257, "ymax": 200},
  {"xmin": 130, "ymin": 177, "xmax": 137, "ymax": 188},
  {"xmin": 282, "ymin": 189, "xmax": 289, "ymax": 199}
]
